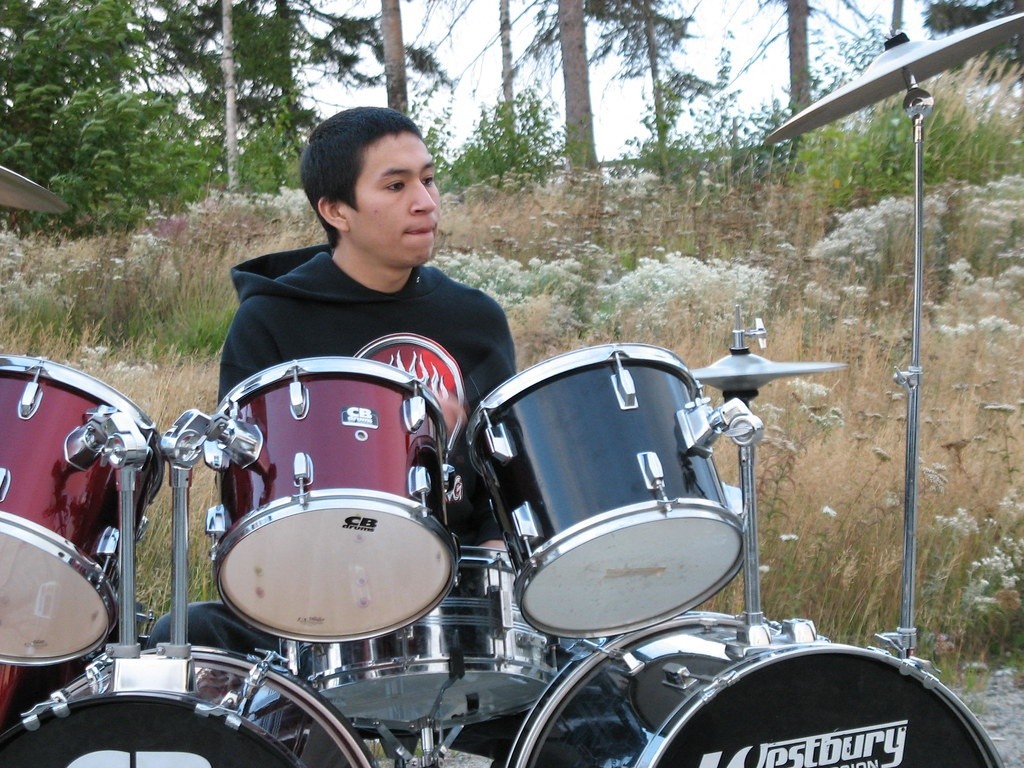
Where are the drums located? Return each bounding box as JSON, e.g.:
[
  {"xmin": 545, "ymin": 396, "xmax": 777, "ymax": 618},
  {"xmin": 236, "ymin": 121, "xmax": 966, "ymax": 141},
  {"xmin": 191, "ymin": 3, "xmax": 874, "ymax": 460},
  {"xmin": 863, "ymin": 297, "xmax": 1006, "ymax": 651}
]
[
  {"xmin": 0, "ymin": 642, "xmax": 377, "ymax": 768},
  {"xmin": 277, "ymin": 545, "xmax": 558, "ymax": 732},
  {"xmin": 502, "ymin": 612, "xmax": 1007, "ymax": 768},
  {"xmin": 465, "ymin": 343, "xmax": 746, "ymax": 637},
  {"xmin": 0, "ymin": 355, "xmax": 166, "ymax": 668},
  {"xmin": 203, "ymin": 355, "xmax": 458, "ymax": 642}
]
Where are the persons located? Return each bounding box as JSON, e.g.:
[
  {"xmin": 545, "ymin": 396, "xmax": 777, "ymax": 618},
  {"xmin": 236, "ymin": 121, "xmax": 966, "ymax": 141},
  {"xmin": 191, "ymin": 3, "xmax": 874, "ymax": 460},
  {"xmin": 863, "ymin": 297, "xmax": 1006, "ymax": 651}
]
[{"xmin": 148, "ymin": 105, "xmax": 517, "ymax": 652}]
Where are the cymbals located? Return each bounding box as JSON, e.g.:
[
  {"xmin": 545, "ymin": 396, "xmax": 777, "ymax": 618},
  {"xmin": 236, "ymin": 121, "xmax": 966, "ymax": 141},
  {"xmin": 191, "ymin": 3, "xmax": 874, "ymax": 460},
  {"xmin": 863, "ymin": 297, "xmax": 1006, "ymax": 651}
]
[
  {"xmin": 759, "ymin": 13, "xmax": 1024, "ymax": 148},
  {"xmin": 0, "ymin": 164, "xmax": 68, "ymax": 214},
  {"xmin": 689, "ymin": 355, "xmax": 850, "ymax": 392}
]
